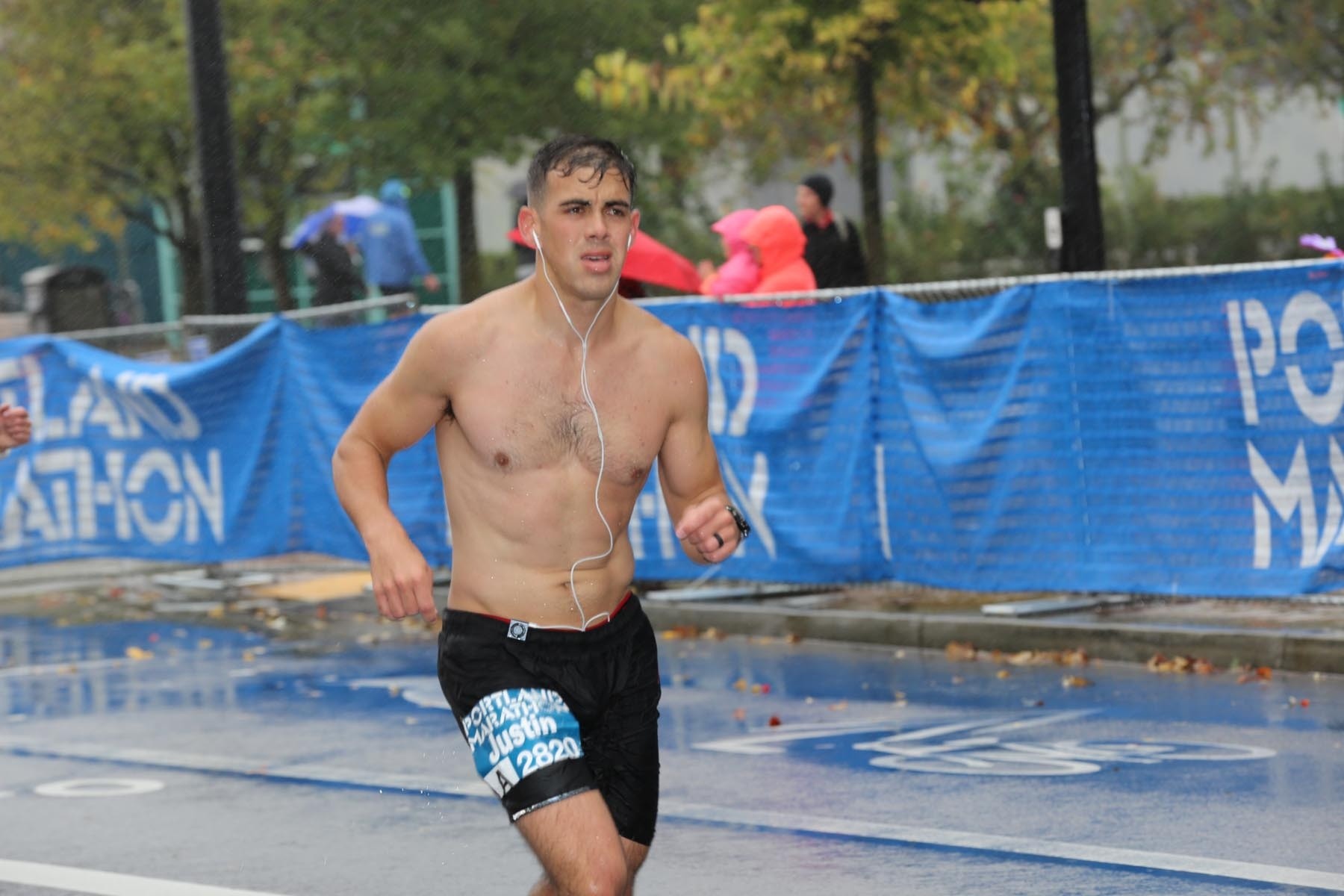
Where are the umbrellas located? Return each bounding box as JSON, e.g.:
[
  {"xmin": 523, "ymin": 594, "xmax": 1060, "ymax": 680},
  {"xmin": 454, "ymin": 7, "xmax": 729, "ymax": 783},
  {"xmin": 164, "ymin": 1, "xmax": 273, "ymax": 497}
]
[
  {"xmin": 509, "ymin": 219, "xmax": 703, "ymax": 295},
  {"xmin": 290, "ymin": 193, "xmax": 383, "ymax": 252}
]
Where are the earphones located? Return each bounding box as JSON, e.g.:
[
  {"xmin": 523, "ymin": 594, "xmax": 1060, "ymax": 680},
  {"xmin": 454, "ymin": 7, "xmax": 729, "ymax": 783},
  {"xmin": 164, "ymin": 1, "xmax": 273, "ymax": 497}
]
[
  {"xmin": 532, "ymin": 230, "xmax": 541, "ymax": 249},
  {"xmin": 627, "ymin": 233, "xmax": 632, "ymax": 249}
]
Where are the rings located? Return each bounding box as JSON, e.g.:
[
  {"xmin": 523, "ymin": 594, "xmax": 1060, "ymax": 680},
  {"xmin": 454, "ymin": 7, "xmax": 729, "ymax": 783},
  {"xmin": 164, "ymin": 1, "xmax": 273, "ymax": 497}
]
[{"xmin": 714, "ymin": 534, "xmax": 724, "ymax": 547}]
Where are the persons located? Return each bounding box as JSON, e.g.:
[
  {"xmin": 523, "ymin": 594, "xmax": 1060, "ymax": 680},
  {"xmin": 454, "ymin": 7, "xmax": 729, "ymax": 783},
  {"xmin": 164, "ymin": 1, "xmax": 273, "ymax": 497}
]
[
  {"xmin": 733, "ymin": 205, "xmax": 816, "ymax": 308},
  {"xmin": 699, "ymin": 209, "xmax": 758, "ymax": 297},
  {"xmin": 355, "ymin": 178, "xmax": 439, "ymax": 314},
  {"xmin": 0, "ymin": 403, "xmax": 31, "ymax": 458},
  {"xmin": 796, "ymin": 173, "xmax": 867, "ymax": 290},
  {"xmin": 332, "ymin": 135, "xmax": 741, "ymax": 896},
  {"xmin": 300, "ymin": 217, "xmax": 367, "ymax": 327}
]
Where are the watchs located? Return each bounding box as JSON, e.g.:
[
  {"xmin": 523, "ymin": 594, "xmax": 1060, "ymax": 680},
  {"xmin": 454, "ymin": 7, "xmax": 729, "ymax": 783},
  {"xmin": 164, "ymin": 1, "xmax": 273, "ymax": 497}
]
[{"xmin": 726, "ymin": 505, "xmax": 749, "ymax": 539}]
